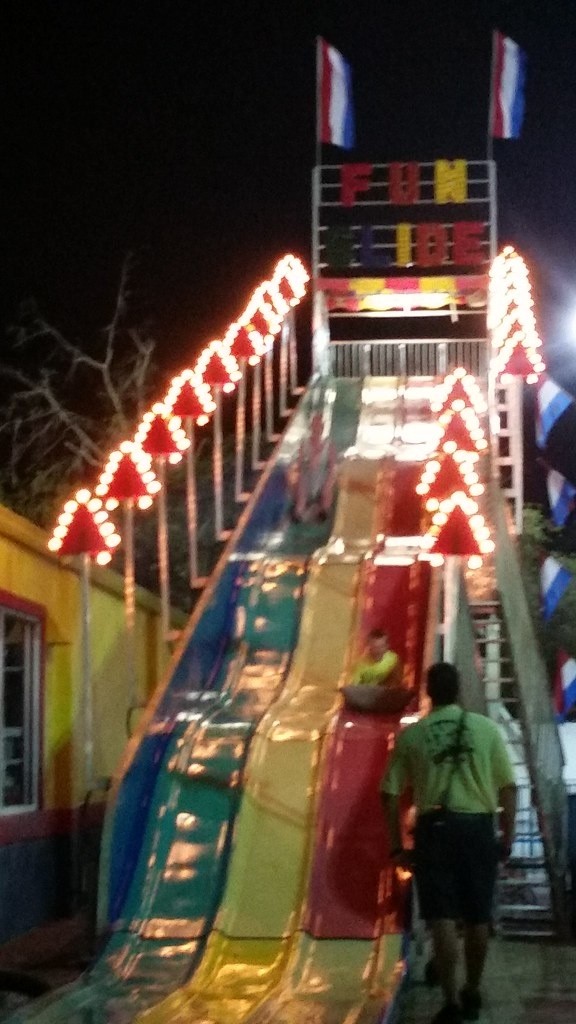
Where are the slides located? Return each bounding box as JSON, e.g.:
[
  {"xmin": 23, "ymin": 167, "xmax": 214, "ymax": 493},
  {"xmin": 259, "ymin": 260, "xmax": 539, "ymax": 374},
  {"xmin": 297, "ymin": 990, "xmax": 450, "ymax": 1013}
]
[{"xmin": 0, "ymin": 373, "xmax": 443, "ymax": 1024}]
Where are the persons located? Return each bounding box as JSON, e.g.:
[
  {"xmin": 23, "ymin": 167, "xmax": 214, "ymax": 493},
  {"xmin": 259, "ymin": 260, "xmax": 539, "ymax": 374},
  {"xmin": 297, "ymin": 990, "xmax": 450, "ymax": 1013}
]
[
  {"xmin": 381, "ymin": 663, "xmax": 518, "ymax": 1024},
  {"xmin": 288, "ymin": 415, "xmax": 337, "ymax": 524},
  {"xmin": 350, "ymin": 629, "xmax": 399, "ymax": 688}
]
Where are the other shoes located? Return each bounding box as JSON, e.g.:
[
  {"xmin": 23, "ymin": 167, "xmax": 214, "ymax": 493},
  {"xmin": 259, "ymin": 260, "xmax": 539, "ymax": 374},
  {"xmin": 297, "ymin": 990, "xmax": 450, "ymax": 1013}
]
[
  {"xmin": 460, "ymin": 986, "xmax": 483, "ymax": 1018},
  {"xmin": 431, "ymin": 1001, "xmax": 463, "ymax": 1024}
]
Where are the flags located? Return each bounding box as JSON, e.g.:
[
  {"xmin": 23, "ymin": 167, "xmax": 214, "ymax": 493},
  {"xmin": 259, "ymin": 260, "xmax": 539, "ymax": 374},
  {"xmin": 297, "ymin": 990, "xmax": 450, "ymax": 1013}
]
[
  {"xmin": 542, "ymin": 459, "xmax": 576, "ymax": 527},
  {"xmin": 488, "ymin": 30, "xmax": 527, "ymax": 139},
  {"xmin": 553, "ymin": 647, "xmax": 576, "ymax": 723},
  {"xmin": 316, "ymin": 35, "xmax": 357, "ymax": 150},
  {"xmin": 538, "ymin": 550, "xmax": 576, "ymax": 621},
  {"xmin": 534, "ymin": 371, "xmax": 576, "ymax": 449}
]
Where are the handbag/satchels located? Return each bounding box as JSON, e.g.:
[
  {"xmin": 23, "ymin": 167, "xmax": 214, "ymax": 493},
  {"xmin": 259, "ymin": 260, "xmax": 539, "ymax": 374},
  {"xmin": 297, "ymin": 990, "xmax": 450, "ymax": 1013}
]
[{"xmin": 412, "ymin": 807, "xmax": 457, "ymax": 854}]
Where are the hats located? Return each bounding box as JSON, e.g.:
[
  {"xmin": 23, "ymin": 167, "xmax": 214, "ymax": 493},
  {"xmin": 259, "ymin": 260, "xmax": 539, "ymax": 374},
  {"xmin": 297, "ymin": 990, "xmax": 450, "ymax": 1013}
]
[{"xmin": 428, "ymin": 663, "xmax": 461, "ymax": 690}]
